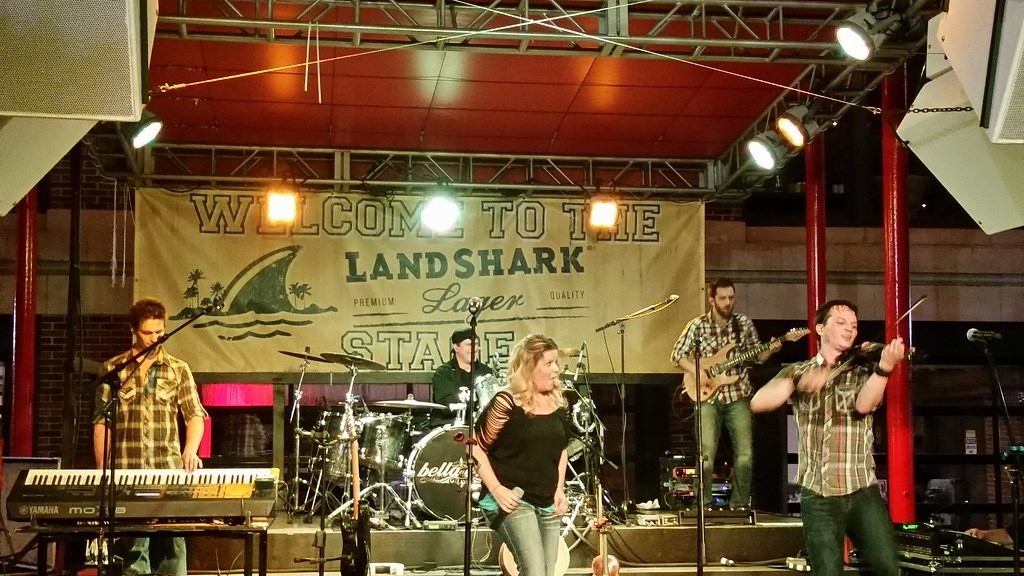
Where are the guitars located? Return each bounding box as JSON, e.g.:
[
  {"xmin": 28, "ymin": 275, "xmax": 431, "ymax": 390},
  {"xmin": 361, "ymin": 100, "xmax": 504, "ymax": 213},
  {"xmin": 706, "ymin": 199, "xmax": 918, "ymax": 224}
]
[{"xmin": 683, "ymin": 328, "xmax": 812, "ymax": 403}]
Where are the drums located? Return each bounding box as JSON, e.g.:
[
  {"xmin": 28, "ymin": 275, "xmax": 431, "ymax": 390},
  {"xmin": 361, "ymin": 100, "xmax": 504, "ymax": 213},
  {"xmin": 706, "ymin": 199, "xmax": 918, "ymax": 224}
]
[
  {"xmin": 311, "ymin": 410, "xmax": 486, "ymax": 525},
  {"xmin": 471, "ymin": 371, "xmax": 509, "ymax": 424}
]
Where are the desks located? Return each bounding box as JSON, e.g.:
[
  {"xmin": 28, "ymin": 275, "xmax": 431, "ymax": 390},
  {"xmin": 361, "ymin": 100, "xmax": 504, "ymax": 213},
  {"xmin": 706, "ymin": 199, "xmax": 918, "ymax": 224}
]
[{"xmin": 14, "ymin": 518, "xmax": 269, "ymax": 576}]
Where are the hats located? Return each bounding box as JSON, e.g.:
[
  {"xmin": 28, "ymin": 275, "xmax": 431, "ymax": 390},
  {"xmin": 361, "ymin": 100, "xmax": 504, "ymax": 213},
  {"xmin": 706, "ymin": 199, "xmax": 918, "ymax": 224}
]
[{"xmin": 451, "ymin": 328, "xmax": 479, "ymax": 353}]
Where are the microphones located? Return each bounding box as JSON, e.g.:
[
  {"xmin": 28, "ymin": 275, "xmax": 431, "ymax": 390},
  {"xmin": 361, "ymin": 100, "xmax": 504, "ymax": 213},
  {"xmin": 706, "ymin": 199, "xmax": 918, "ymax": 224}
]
[
  {"xmin": 967, "ymin": 327, "xmax": 1002, "ymax": 342},
  {"xmin": 361, "ymin": 397, "xmax": 369, "ymax": 412},
  {"xmin": 469, "ymin": 297, "xmax": 482, "ymax": 314},
  {"xmin": 670, "ymin": 294, "xmax": 680, "ymax": 301},
  {"xmin": 574, "ymin": 342, "xmax": 585, "ymax": 380}
]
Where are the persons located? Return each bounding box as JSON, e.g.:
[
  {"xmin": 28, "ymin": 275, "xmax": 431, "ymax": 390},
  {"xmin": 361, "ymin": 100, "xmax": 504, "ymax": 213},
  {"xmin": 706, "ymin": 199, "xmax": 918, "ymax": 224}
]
[
  {"xmin": 749, "ymin": 298, "xmax": 915, "ymax": 576},
  {"xmin": 92, "ymin": 297, "xmax": 208, "ymax": 576},
  {"xmin": 466, "ymin": 334, "xmax": 572, "ymax": 576},
  {"xmin": 670, "ymin": 276, "xmax": 781, "ymax": 511},
  {"xmin": 428, "ymin": 328, "xmax": 494, "ymax": 431},
  {"xmin": 964, "ymin": 518, "xmax": 1024, "ymax": 551}
]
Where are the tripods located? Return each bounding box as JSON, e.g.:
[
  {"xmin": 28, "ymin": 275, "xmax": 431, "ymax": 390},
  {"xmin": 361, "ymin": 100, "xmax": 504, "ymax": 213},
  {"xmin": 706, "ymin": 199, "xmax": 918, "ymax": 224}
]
[
  {"xmin": 595, "ymin": 298, "xmax": 673, "ymax": 526},
  {"xmin": 277, "ymin": 357, "xmax": 423, "ymax": 530}
]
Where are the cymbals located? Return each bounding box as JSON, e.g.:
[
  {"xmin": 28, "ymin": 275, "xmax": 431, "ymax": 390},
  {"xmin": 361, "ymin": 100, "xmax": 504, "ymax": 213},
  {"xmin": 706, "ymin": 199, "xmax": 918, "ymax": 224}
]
[
  {"xmin": 559, "ymin": 348, "xmax": 581, "ymax": 358},
  {"xmin": 278, "ymin": 350, "xmax": 388, "ymax": 370},
  {"xmin": 372, "ymin": 398, "xmax": 448, "ymax": 410}
]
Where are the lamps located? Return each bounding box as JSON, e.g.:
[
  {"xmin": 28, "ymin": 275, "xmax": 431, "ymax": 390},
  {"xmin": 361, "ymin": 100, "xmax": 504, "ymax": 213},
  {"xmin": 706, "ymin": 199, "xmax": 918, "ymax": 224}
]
[
  {"xmin": 120, "ymin": 107, "xmax": 163, "ymax": 148},
  {"xmin": 833, "ymin": 9, "xmax": 898, "ymax": 61},
  {"xmin": 778, "ymin": 104, "xmax": 816, "ymax": 146},
  {"xmin": 747, "ymin": 130, "xmax": 789, "ymax": 171}
]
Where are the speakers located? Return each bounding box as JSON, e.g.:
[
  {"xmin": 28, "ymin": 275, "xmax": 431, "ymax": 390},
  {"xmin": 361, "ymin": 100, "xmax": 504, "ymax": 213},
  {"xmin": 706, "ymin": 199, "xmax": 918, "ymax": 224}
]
[
  {"xmin": 0, "ymin": 0, "xmax": 158, "ymax": 123},
  {"xmin": 0, "ymin": 456, "xmax": 89, "ymax": 575},
  {"xmin": 937, "ymin": 0, "xmax": 1024, "ymax": 145}
]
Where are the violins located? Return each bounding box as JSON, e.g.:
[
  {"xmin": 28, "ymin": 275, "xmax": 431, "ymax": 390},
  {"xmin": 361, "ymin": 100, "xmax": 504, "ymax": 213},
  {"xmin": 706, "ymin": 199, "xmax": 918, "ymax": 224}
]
[{"xmin": 839, "ymin": 341, "xmax": 921, "ymax": 363}]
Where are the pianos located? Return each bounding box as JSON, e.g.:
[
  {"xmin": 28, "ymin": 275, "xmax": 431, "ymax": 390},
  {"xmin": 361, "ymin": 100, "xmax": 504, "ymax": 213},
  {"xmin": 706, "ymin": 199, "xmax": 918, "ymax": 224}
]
[{"xmin": 6, "ymin": 467, "xmax": 279, "ymax": 576}]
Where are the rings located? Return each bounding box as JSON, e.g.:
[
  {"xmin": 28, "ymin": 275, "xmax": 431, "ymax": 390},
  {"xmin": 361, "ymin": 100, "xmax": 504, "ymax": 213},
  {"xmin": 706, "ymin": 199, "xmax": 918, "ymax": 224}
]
[{"xmin": 510, "ymin": 501, "xmax": 513, "ymax": 505}]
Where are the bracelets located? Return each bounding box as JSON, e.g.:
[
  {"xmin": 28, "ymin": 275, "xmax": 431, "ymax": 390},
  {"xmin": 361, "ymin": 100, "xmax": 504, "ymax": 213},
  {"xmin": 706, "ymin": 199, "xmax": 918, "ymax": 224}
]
[
  {"xmin": 793, "ymin": 375, "xmax": 801, "ymax": 387},
  {"xmin": 873, "ymin": 363, "xmax": 891, "ymax": 377}
]
